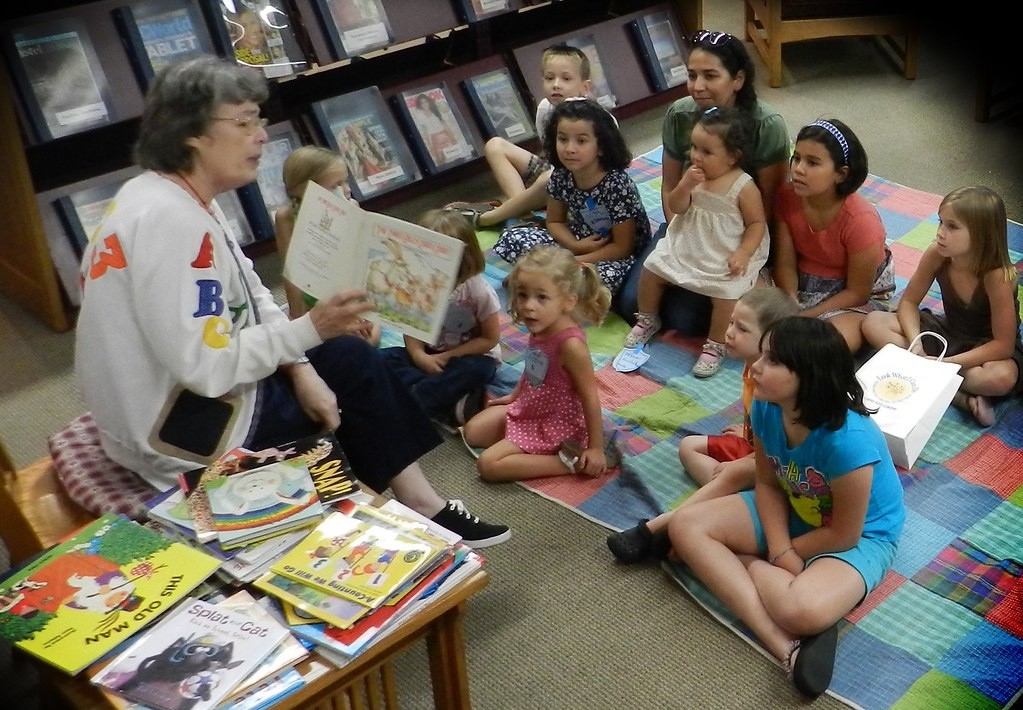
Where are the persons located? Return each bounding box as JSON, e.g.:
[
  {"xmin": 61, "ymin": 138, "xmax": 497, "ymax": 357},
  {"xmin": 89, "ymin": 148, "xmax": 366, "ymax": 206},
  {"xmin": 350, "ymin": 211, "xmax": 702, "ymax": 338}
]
[
  {"xmin": 619, "ymin": 29, "xmax": 790, "ymax": 329},
  {"xmin": 229, "ymin": 0, "xmax": 268, "ymax": 53},
  {"xmin": 445, "ymin": 46, "xmax": 619, "ymax": 228},
  {"xmin": 380, "ymin": 209, "xmax": 501, "ymax": 422},
  {"xmin": 862, "ymin": 184, "xmax": 1023, "ymax": 426},
  {"xmin": 275, "ymin": 144, "xmax": 360, "ymax": 317},
  {"xmin": 607, "ymin": 286, "xmax": 800, "ymax": 564},
  {"xmin": 492, "ymin": 97, "xmax": 654, "ymax": 288},
  {"xmin": 464, "ymin": 245, "xmax": 614, "ymax": 481},
  {"xmin": 622, "ymin": 106, "xmax": 772, "ymax": 374},
  {"xmin": 73, "ymin": 55, "xmax": 513, "ymax": 548},
  {"xmin": 772, "ymin": 118, "xmax": 897, "ymax": 352},
  {"xmin": 416, "ymin": 94, "xmax": 458, "ymax": 165},
  {"xmin": 669, "ymin": 315, "xmax": 906, "ymax": 695}
]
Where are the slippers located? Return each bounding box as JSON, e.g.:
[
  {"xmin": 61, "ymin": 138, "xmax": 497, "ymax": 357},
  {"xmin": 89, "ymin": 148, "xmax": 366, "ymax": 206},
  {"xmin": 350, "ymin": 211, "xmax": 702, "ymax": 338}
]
[{"xmin": 606, "ymin": 518, "xmax": 664, "ymax": 562}]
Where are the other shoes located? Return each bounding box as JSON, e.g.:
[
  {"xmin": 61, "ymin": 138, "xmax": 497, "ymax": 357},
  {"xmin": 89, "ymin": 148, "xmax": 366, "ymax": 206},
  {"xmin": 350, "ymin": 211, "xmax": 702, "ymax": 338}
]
[
  {"xmin": 623, "ymin": 313, "xmax": 662, "ymax": 347},
  {"xmin": 965, "ymin": 394, "xmax": 995, "ymax": 427},
  {"xmin": 692, "ymin": 338, "xmax": 725, "ymax": 379},
  {"xmin": 785, "ymin": 622, "xmax": 838, "ymax": 694},
  {"xmin": 454, "ymin": 388, "xmax": 490, "ymax": 423}
]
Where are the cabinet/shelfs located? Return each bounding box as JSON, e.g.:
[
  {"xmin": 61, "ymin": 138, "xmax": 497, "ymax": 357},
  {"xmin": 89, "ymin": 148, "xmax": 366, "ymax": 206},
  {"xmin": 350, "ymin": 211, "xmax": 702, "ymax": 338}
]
[{"xmin": 0, "ymin": 0, "xmax": 703, "ymax": 333}]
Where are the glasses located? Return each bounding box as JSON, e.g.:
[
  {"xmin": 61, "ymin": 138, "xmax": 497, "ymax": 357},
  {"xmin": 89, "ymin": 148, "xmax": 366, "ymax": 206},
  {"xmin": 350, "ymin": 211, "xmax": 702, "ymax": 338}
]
[
  {"xmin": 212, "ymin": 117, "xmax": 270, "ymax": 134},
  {"xmin": 690, "ymin": 29, "xmax": 742, "ymax": 69}
]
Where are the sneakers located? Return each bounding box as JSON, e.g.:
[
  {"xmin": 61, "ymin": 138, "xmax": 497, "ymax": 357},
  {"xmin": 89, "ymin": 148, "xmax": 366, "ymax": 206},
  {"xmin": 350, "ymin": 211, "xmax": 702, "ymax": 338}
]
[{"xmin": 431, "ymin": 501, "xmax": 514, "ymax": 549}]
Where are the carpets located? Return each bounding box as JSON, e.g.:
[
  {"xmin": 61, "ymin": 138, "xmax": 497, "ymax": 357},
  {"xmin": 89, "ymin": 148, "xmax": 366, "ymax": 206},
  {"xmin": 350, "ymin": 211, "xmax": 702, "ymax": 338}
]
[{"xmin": 383, "ymin": 145, "xmax": 1023, "ymax": 710}]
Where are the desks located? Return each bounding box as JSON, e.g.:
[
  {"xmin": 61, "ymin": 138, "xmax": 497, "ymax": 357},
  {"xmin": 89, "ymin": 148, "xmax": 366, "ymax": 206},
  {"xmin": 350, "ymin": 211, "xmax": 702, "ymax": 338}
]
[
  {"xmin": 742, "ymin": 0, "xmax": 919, "ymax": 88},
  {"xmin": 0, "ymin": 481, "xmax": 489, "ymax": 710}
]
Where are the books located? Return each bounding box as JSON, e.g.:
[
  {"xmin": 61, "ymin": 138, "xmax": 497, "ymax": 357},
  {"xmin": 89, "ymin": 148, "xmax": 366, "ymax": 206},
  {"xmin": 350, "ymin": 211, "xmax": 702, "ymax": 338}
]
[
  {"xmin": 0, "ymin": 0, "xmax": 690, "ymax": 248},
  {"xmin": 281, "ymin": 179, "xmax": 466, "ymax": 345},
  {"xmin": 0, "ymin": 429, "xmax": 486, "ymax": 710}
]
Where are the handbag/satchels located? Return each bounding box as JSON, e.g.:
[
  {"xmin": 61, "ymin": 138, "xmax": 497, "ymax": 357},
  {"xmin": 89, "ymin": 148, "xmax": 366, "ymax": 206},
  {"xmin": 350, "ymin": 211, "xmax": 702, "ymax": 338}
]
[{"xmin": 848, "ymin": 329, "xmax": 964, "ymax": 470}]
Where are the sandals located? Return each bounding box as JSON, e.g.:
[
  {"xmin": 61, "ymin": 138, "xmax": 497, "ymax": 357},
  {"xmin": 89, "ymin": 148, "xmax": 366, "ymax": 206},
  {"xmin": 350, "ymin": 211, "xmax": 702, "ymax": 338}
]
[
  {"xmin": 560, "ymin": 440, "xmax": 615, "ymax": 475},
  {"xmin": 444, "ymin": 199, "xmax": 504, "ymax": 231}
]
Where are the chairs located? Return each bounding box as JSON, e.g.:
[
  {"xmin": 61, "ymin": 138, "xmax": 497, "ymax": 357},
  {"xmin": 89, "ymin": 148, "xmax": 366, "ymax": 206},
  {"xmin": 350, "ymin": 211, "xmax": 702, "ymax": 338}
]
[{"xmin": 0, "ymin": 414, "xmax": 157, "ymax": 564}]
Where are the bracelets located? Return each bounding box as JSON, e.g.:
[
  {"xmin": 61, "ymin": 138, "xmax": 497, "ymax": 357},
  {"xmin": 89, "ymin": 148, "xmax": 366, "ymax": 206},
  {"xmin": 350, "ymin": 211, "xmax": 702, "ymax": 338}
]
[{"xmin": 769, "ymin": 547, "xmax": 794, "ymax": 564}]
